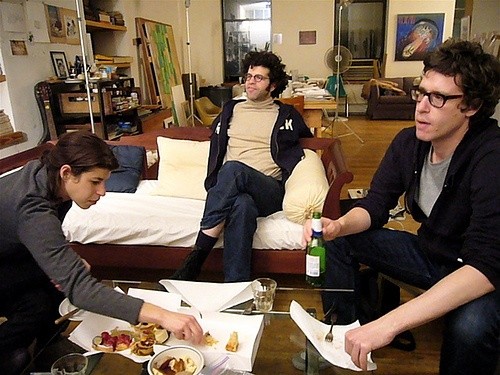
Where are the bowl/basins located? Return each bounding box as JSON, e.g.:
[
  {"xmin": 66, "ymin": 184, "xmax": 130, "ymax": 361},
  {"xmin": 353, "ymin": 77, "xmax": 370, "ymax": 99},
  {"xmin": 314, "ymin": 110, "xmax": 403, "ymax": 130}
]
[{"xmin": 147, "ymin": 346, "xmax": 204, "ymax": 375}]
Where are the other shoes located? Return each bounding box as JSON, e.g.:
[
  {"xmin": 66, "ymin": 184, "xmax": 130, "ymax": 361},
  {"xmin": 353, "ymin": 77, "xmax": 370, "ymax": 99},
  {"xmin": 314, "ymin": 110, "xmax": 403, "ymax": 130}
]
[{"xmin": 292, "ymin": 346, "xmax": 332, "ymax": 372}]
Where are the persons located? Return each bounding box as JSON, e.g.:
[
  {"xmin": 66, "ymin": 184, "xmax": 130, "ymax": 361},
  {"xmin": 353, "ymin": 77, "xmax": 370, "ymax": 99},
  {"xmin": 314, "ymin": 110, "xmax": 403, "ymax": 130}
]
[
  {"xmin": 302, "ymin": 40, "xmax": 500, "ymax": 375},
  {"xmin": 65, "ymin": 18, "xmax": 76, "ymax": 35},
  {"xmin": 0, "ymin": 130, "xmax": 203, "ymax": 348},
  {"xmin": 166, "ymin": 50, "xmax": 316, "ymax": 283}
]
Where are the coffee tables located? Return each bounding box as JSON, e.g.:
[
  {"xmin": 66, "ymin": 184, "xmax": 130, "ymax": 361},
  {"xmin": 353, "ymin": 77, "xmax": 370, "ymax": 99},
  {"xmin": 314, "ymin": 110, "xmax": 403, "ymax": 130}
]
[{"xmin": 15, "ymin": 279, "xmax": 354, "ymax": 375}]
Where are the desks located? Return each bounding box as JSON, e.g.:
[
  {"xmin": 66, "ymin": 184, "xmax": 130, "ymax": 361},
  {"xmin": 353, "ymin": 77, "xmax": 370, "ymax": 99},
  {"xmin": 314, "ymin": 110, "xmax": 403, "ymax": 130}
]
[{"xmin": 232, "ymin": 94, "xmax": 337, "ymax": 159}]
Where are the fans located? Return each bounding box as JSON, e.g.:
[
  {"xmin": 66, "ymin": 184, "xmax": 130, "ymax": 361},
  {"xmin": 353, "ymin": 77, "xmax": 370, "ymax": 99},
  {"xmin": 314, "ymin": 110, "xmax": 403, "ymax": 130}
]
[{"xmin": 323, "ymin": 45, "xmax": 353, "ymax": 74}]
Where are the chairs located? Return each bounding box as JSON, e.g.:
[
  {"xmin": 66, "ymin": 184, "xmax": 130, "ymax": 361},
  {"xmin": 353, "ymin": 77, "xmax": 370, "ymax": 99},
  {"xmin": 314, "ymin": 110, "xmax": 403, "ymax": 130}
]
[
  {"xmin": 322, "ymin": 75, "xmax": 349, "ymax": 118},
  {"xmin": 358, "ymin": 191, "xmax": 428, "ymax": 319},
  {"xmin": 195, "ymin": 96, "xmax": 223, "ymax": 128}
]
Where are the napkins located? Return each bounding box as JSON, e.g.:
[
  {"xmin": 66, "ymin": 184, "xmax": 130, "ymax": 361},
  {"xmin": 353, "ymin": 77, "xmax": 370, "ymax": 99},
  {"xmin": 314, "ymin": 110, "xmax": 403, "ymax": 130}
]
[
  {"xmin": 159, "ymin": 277, "xmax": 263, "ymax": 318},
  {"xmin": 290, "ymin": 298, "xmax": 377, "ymax": 372}
]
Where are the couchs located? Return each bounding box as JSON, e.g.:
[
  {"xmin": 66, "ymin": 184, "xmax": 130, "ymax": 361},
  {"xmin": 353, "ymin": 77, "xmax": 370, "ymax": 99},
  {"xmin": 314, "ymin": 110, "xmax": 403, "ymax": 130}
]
[
  {"xmin": 364, "ymin": 77, "xmax": 420, "ymax": 121},
  {"xmin": 45, "ymin": 127, "xmax": 354, "ymax": 276}
]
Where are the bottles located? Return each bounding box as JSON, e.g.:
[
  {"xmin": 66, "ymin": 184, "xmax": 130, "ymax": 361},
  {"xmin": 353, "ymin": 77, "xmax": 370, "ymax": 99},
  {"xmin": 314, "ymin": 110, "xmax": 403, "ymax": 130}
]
[
  {"xmin": 106, "ymin": 67, "xmax": 112, "ymax": 81},
  {"xmin": 305, "ymin": 212, "xmax": 326, "ymax": 288},
  {"xmin": 68, "ymin": 60, "xmax": 90, "ymax": 79}
]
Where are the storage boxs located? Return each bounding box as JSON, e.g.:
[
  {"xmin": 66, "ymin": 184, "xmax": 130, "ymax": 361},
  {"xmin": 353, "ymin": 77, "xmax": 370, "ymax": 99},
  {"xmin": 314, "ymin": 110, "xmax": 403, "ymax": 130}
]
[
  {"xmin": 94, "ymin": 57, "xmax": 133, "ymax": 64},
  {"xmin": 98, "ymin": 14, "xmax": 110, "ymax": 23},
  {"xmin": 59, "ymin": 91, "xmax": 112, "ymax": 114}
]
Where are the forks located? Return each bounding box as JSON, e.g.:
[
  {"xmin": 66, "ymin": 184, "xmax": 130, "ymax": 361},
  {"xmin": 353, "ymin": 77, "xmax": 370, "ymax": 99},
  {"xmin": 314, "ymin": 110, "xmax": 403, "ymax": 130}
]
[{"xmin": 325, "ymin": 313, "xmax": 336, "ymax": 341}]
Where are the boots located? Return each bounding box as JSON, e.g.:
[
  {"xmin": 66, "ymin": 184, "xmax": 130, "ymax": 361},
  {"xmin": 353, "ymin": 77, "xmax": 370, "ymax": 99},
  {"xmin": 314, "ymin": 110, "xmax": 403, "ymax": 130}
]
[{"xmin": 158, "ymin": 243, "xmax": 209, "ymax": 291}]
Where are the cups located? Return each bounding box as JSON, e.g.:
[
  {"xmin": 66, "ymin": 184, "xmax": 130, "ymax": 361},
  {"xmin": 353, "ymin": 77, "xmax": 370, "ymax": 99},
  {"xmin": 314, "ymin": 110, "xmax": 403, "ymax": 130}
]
[
  {"xmin": 251, "ymin": 277, "xmax": 276, "ymax": 312},
  {"xmin": 51, "ymin": 353, "xmax": 88, "ymax": 375},
  {"xmin": 94, "ymin": 71, "xmax": 103, "ymax": 77}
]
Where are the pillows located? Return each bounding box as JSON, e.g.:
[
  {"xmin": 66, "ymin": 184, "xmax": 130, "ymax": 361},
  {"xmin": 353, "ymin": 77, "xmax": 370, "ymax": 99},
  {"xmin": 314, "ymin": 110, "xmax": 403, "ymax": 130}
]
[
  {"xmin": 104, "ymin": 145, "xmax": 147, "ymax": 193},
  {"xmin": 282, "ymin": 149, "xmax": 330, "ymax": 226},
  {"xmin": 153, "ymin": 136, "xmax": 210, "ymax": 202}
]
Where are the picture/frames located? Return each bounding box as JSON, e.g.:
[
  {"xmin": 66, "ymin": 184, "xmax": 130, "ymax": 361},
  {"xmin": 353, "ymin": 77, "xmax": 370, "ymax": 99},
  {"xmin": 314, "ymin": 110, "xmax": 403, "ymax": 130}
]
[{"xmin": 49, "ymin": 51, "xmax": 70, "ymax": 80}]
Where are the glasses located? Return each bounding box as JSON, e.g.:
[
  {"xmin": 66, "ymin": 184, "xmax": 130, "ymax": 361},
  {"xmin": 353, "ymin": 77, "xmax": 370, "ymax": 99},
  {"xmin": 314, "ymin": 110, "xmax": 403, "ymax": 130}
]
[
  {"xmin": 408, "ymin": 85, "xmax": 464, "ymax": 108},
  {"xmin": 244, "ymin": 74, "xmax": 269, "ymax": 83}
]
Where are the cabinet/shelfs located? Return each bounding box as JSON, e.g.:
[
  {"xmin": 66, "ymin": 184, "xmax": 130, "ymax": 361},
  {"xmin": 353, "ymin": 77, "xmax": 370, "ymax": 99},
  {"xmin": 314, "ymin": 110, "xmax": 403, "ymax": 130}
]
[
  {"xmin": 86, "ymin": 19, "xmax": 130, "ymax": 68},
  {"xmin": 198, "ymin": 86, "xmax": 233, "ymax": 107},
  {"xmin": 0, "ymin": 74, "xmax": 29, "ymax": 150},
  {"xmin": 34, "ymin": 77, "xmax": 142, "ymax": 145}
]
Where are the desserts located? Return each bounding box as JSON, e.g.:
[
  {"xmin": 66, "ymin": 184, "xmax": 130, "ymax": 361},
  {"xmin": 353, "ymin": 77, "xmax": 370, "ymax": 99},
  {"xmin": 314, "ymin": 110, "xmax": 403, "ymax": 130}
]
[
  {"xmin": 224, "ymin": 332, "xmax": 239, "ymax": 352},
  {"xmin": 91, "ymin": 321, "xmax": 162, "ymax": 357}
]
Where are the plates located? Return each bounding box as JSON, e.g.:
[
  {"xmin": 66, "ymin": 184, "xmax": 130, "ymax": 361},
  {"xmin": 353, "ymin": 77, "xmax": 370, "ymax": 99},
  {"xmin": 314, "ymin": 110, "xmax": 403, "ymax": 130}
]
[{"xmin": 58, "ymin": 298, "xmax": 90, "ymax": 321}]
